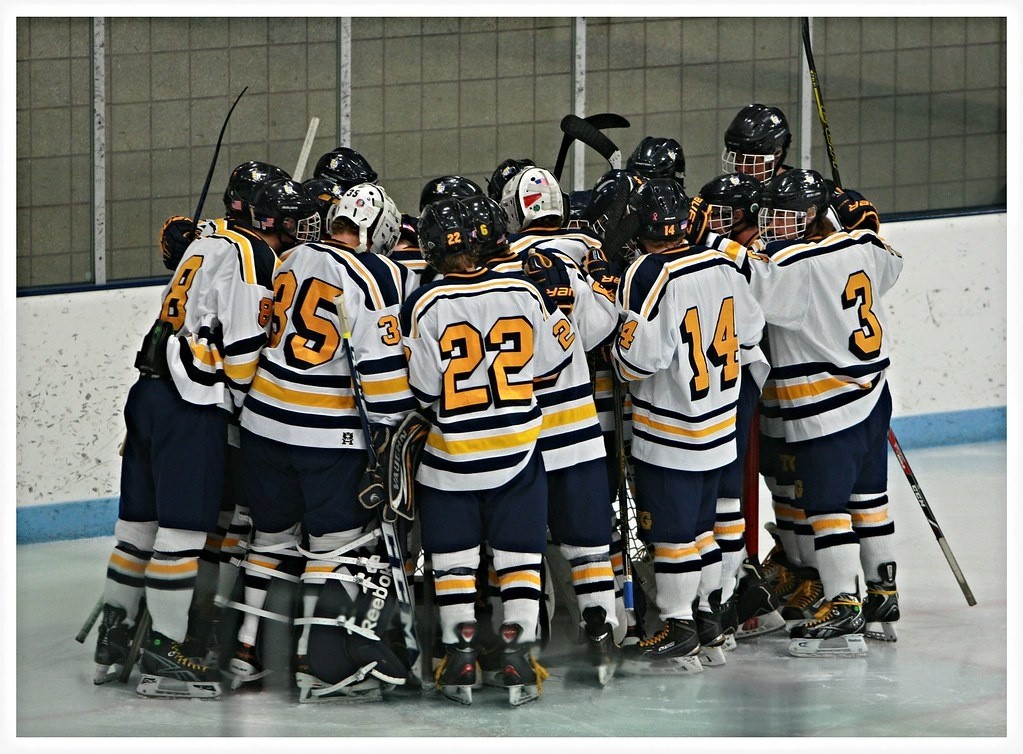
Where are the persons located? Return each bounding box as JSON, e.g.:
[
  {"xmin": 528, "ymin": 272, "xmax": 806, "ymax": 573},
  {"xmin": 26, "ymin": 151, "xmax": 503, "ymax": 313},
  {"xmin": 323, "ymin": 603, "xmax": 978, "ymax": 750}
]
[{"xmin": 92, "ymin": 102, "xmax": 899, "ymax": 708}]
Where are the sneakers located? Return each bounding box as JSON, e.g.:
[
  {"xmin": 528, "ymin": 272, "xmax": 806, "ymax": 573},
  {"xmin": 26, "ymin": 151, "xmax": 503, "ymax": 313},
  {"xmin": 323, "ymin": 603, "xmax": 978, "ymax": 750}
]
[{"xmin": 75, "ymin": 522, "xmax": 900, "ymax": 705}]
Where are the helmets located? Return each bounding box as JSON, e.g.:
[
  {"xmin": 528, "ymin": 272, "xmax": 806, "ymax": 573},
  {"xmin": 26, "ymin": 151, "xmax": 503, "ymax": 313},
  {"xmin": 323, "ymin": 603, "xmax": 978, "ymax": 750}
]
[
  {"xmin": 303, "ymin": 179, "xmax": 344, "ymax": 240},
  {"xmin": 461, "ymin": 195, "xmax": 509, "ymax": 256},
  {"xmin": 570, "ymin": 170, "xmax": 637, "ymax": 263},
  {"xmin": 759, "ymin": 168, "xmax": 829, "ymax": 242},
  {"xmin": 314, "ymin": 146, "xmax": 378, "ymax": 196},
  {"xmin": 417, "ymin": 198, "xmax": 477, "ymax": 272},
  {"xmin": 223, "ymin": 161, "xmax": 292, "ymax": 217},
  {"xmin": 400, "ymin": 212, "xmax": 419, "ymax": 247},
  {"xmin": 249, "ymin": 178, "xmax": 322, "ymax": 242},
  {"xmin": 626, "ymin": 137, "xmax": 686, "ymax": 187},
  {"xmin": 699, "ymin": 172, "xmax": 762, "ymax": 237},
  {"xmin": 330, "ymin": 183, "xmax": 402, "ymax": 256},
  {"xmin": 725, "ymin": 103, "xmax": 791, "ymax": 184},
  {"xmin": 626, "ymin": 177, "xmax": 689, "ymax": 241},
  {"xmin": 499, "ymin": 165, "xmax": 564, "ymax": 234},
  {"xmin": 419, "ymin": 175, "xmax": 483, "ymax": 214},
  {"xmin": 488, "ymin": 159, "xmax": 537, "ymax": 201}
]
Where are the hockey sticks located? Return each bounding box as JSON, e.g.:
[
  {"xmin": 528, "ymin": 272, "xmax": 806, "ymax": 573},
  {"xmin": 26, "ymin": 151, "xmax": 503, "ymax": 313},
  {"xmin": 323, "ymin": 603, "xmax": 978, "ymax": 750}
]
[
  {"xmin": 554, "ymin": 113, "xmax": 634, "ymax": 190},
  {"xmin": 335, "ymin": 300, "xmax": 451, "ymax": 695},
  {"xmin": 801, "ymin": 15, "xmax": 840, "ymax": 187},
  {"xmin": 607, "ymin": 331, "xmax": 636, "ymax": 618},
  {"xmin": 881, "ymin": 416, "xmax": 977, "ymax": 607}
]
